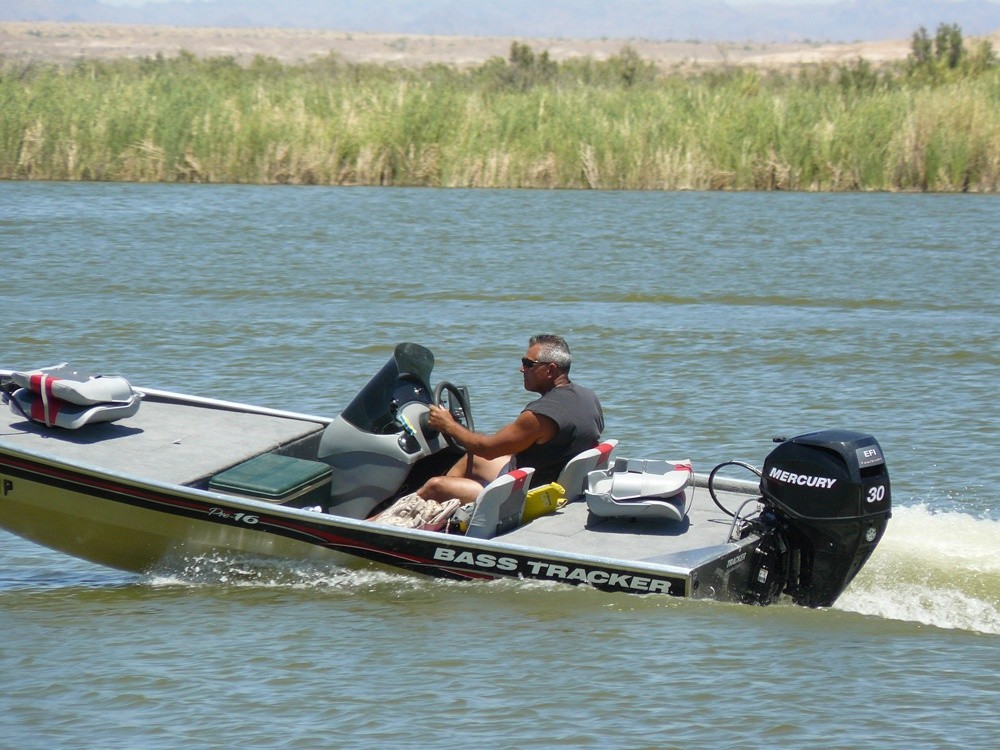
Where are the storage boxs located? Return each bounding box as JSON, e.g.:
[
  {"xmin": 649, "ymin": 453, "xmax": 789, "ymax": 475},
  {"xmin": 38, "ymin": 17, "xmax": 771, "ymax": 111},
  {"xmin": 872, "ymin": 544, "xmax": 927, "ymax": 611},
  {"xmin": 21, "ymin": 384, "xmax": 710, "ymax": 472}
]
[{"xmin": 209, "ymin": 453, "xmax": 333, "ymax": 515}]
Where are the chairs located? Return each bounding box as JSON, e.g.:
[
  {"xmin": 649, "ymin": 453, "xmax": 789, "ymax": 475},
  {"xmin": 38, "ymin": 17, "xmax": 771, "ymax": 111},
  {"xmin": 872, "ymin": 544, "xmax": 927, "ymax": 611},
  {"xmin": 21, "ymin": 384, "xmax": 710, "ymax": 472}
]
[
  {"xmin": 558, "ymin": 439, "xmax": 620, "ymax": 504},
  {"xmin": 466, "ymin": 468, "xmax": 535, "ymax": 541}
]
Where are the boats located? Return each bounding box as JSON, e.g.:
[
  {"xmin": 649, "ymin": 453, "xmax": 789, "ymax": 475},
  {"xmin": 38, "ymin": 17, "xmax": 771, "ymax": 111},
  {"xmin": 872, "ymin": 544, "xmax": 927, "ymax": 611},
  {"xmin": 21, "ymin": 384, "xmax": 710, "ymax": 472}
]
[{"xmin": 0, "ymin": 366, "xmax": 894, "ymax": 615}]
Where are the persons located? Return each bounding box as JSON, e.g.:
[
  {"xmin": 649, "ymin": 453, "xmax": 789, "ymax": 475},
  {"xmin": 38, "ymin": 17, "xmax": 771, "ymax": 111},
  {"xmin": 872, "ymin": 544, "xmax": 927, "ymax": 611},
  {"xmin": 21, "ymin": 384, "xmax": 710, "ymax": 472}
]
[{"xmin": 365, "ymin": 335, "xmax": 605, "ymax": 521}]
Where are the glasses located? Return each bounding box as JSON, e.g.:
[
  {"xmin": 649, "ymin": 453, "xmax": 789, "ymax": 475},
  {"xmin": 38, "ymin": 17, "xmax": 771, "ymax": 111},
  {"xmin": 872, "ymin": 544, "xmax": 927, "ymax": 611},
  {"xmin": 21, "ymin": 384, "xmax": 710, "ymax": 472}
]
[{"xmin": 521, "ymin": 357, "xmax": 558, "ymax": 369}]
